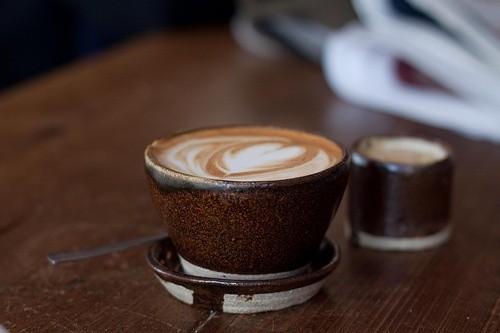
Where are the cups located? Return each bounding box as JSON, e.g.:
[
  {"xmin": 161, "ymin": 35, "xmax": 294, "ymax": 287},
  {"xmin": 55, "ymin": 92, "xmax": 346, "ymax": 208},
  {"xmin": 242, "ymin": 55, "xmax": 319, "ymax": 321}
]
[
  {"xmin": 348, "ymin": 135, "xmax": 456, "ymax": 250},
  {"xmin": 143, "ymin": 125, "xmax": 350, "ymax": 279}
]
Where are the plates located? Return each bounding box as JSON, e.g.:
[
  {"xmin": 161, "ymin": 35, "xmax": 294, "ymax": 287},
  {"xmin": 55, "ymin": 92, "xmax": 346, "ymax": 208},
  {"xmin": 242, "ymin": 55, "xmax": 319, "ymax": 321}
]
[{"xmin": 144, "ymin": 235, "xmax": 340, "ymax": 313}]
